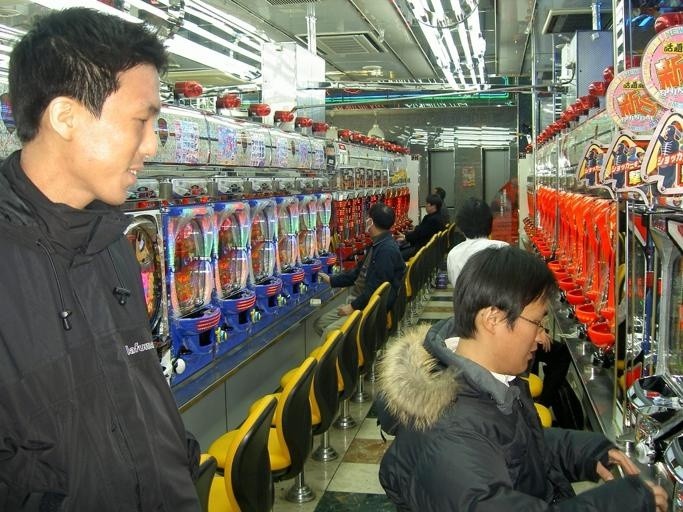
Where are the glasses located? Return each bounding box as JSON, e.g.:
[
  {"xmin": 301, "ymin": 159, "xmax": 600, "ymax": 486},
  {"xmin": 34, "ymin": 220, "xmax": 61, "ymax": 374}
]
[{"xmin": 516, "ymin": 312, "xmax": 552, "ymax": 334}]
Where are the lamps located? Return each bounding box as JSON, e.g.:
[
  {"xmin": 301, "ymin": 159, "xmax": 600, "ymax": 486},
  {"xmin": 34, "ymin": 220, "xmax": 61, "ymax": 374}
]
[{"xmin": 366, "ymin": 110, "xmax": 385, "ymax": 141}]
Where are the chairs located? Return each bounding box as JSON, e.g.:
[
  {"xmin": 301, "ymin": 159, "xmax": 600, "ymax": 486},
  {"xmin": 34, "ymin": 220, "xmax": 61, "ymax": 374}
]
[
  {"xmin": 519, "ymin": 371, "xmax": 543, "ymax": 399},
  {"xmin": 310, "ymin": 281, "xmax": 395, "ymax": 464},
  {"xmin": 532, "ymin": 402, "xmax": 552, "ymax": 428},
  {"xmin": 404, "ymin": 223, "xmax": 456, "ymax": 302},
  {"xmin": 199, "ymin": 357, "xmax": 318, "ymax": 511}
]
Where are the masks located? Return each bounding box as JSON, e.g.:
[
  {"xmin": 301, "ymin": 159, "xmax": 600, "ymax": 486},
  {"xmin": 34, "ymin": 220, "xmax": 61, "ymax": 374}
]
[{"xmin": 365, "ymin": 217, "xmax": 374, "ymax": 233}]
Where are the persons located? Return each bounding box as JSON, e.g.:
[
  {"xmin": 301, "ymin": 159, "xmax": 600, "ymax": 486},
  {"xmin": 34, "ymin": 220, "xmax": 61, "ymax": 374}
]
[
  {"xmin": 312, "ymin": 203, "xmax": 408, "ymax": 346},
  {"xmin": 432, "ymin": 187, "xmax": 451, "ymax": 223},
  {"xmin": 374, "ymin": 245, "xmax": 669, "ymax": 512},
  {"xmin": 1, "ymin": 6, "xmax": 203, "ymax": 511},
  {"xmin": 500, "ymin": 177, "xmax": 518, "ymax": 214},
  {"xmin": 395, "ymin": 194, "xmax": 446, "ymax": 262},
  {"xmin": 446, "ymin": 198, "xmax": 513, "ymax": 289}
]
